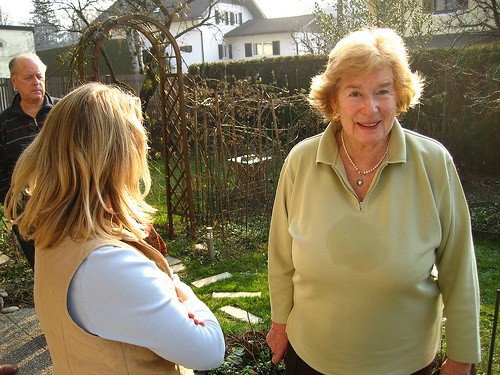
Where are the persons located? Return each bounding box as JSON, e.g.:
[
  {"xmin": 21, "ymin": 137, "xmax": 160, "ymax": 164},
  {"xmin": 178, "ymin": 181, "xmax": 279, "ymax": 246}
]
[
  {"xmin": 6, "ymin": 81, "xmax": 226, "ymax": 375},
  {"xmin": 266, "ymin": 26, "xmax": 481, "ymax": 375},
  {"xmin": 0, "ymin": 54, "xmax": 64, "ymax": 273}
]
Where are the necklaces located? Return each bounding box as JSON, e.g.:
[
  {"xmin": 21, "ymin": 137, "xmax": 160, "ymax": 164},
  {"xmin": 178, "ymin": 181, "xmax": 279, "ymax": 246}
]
[{"xmin": 341, "ymin": 127, "xmax": 393, "ymax": 186}]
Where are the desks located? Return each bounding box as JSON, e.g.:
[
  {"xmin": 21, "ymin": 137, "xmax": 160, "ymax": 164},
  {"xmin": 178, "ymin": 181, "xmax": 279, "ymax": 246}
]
[{"xmin": 228, "ymin": 154, "xmax": 272, "ymax": 201}]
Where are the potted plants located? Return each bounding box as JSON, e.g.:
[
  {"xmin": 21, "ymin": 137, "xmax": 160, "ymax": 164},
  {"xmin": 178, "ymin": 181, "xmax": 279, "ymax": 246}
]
[{"xmin": 470, "ymin": 206, "xmax": 500, "ymax": 241}]
